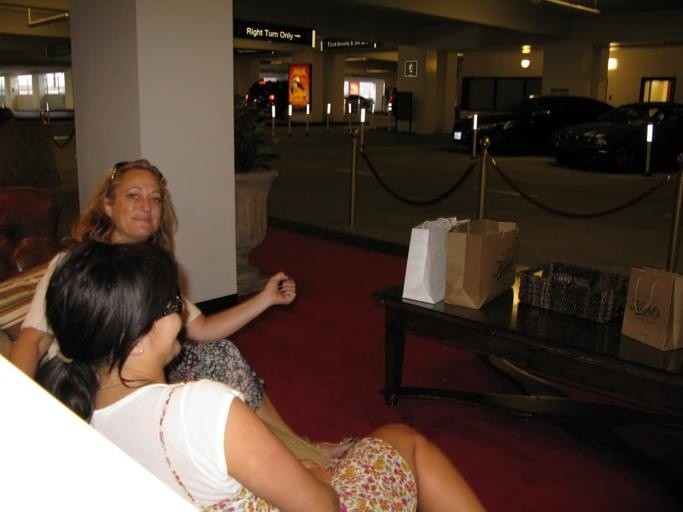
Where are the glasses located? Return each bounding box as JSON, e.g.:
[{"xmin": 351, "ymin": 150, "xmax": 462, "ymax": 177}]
[
  {"xmin": 156, "ymin": 291, "xmax": 183, "ymax": 318},
  {"xmin": 110, "ymin": 159, "xmax": 166, "ymax": 188}
]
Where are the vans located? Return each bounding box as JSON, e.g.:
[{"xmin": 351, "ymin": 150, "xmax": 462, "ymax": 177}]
[{"xmin": 242, "ymin": 74, "xmax": 288, "ymax": 118}]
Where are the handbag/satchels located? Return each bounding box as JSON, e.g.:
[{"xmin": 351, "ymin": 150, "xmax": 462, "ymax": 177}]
[
  {"xmin": 620, "ymin": 264, "xmax": 683, "ymax": 352},
  {"xmin": 401, "ymin": 215, "xmax": 473, "ymax": 306},
  {"xmin": 442, "ymin": 217, "xmax": 521, "ymax": 311}
]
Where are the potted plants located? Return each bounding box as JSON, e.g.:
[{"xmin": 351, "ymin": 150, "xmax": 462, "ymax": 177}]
[{"xmin": 234, "ymin": 94, "xmax": 278, "ymax": 297}]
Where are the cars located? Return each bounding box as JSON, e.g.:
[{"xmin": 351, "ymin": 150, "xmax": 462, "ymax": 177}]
[
  {"xmin": 343, "ymin": 93, "xmax": 374, "ymax": 114},
  {"xmin": 445, "ymin": 90, "xmax": 618, "ymax": 159},
  {"xmin": 544, "ymin": 95, "xmax": 681, "ymax": 176}
]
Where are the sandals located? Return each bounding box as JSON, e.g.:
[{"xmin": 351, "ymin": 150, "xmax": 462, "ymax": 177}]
[{"xmin": 313, "ymin": 435, "xmax": 359, "ymax": 460}]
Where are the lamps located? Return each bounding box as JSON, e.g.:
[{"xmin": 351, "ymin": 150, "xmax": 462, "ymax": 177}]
[
  {"xmin": 643, "ymin": 121, "xmax": 654, "ymax": 177},
  {"xmin": 471, "ymin": 112, "xmax": 478, "ymax": 160},
  {"xmin": 269, "ymin": 98, "xmax": 394, "ymax": 150}
]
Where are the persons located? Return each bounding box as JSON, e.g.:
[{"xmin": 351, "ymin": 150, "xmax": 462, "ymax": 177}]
[
  {"xmin": 35, "ymin": 243, "xmax": 485, "ymax": 512},
  {"xmin": 0, "ymin": 106, "xmax": 21, "ymax": 187},
  {"xmin": 9, "ymin": 158, "xmax": 358, "ymax": 467},
  {"xmin": 233, "ymin": 116, "xmax": 260, "ymax": 174}
]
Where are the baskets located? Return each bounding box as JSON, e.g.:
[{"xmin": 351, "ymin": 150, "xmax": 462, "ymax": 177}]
[{"xmin": 514, "ymin": 260, "xmax": 628, "ymax": 324}]
